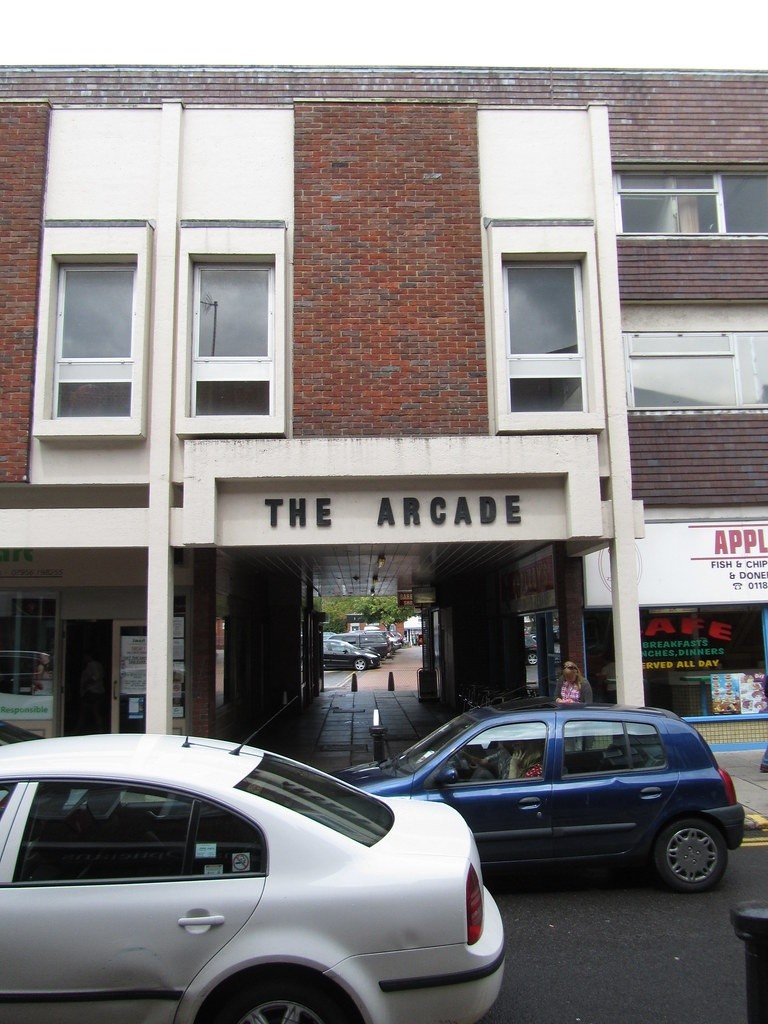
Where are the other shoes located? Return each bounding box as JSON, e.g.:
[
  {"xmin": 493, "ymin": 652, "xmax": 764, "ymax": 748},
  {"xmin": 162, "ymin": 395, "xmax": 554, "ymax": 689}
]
[{"xmin": 760, "ymin": 763, "xmax": 768, "ymax": 771}]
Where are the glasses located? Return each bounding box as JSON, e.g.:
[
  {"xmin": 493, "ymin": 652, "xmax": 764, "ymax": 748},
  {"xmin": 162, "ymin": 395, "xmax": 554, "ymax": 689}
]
[{"xmin": 560, "ymin": 665, "xmax": 578, "ymax": 674}]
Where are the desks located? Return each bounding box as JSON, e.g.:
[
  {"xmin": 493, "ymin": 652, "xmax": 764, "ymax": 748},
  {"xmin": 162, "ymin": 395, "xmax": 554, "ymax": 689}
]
[
  {"xmin": 604, "ymin": 678, "xmax": 648, "ymax": 684},
  {"xmin": 679, "ymin": 676, "xmax": 712, "ymax": 716}
]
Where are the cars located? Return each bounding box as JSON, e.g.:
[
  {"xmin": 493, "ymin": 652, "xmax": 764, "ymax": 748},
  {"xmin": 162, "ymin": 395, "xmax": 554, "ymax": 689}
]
[
  {"xmin": 323, "ymin": 639, "xmax": 379, "ymax": 670},
  {"xmin": 326, "ymin": 693, "xmax": 745, "ymax": 893},
  {"xmin": 524, "ymin": 625, "xmax": 560, "ymax": 665},
  {"xmin": 0, "ymin": 696, "xmax": 505, "ymax": 1023}
]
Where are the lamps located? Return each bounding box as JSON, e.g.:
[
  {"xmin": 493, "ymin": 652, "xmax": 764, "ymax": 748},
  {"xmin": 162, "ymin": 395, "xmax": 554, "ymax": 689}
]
[{"xmin": 377, "ymin": 555, "xmax": 386, "ymax": 568}]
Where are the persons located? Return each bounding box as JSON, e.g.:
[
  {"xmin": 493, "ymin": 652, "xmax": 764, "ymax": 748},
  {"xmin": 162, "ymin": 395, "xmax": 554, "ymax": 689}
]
[
  {"xmin": 553, "ymin": 661, "xmax": 593, "ymax": 704},
  {"xmin": 470, "ymin": 737, "xmax": 544, "ymax": 781},
  {"xmin": 760, "ymin": 675, "xmax": 768, "ymax": 772}
]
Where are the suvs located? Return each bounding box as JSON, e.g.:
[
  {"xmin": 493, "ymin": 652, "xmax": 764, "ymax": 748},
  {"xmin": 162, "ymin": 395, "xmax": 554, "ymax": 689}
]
[{"xmin": 323, "ymin": 629, "xmax": 405, "ymax": 658}]
